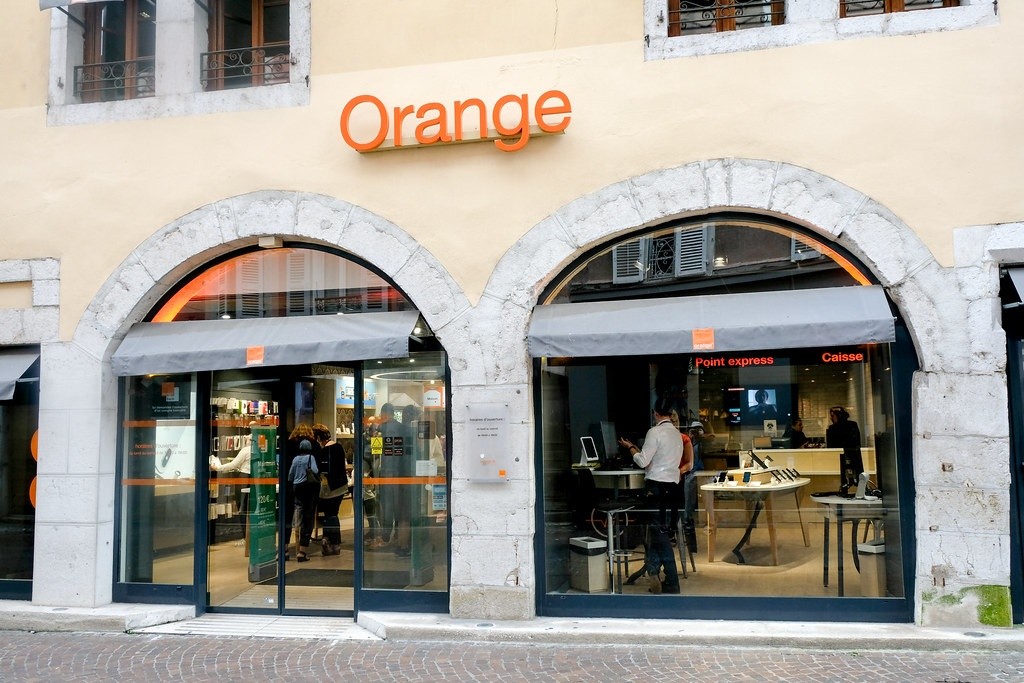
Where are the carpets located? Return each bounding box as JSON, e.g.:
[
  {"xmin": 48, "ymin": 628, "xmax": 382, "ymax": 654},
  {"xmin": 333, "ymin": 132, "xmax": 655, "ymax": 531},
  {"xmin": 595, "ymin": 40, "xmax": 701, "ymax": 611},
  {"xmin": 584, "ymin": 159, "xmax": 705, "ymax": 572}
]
[{"xmin": 255, "ymin": 566, "xmax": 410, "ymax": 588}]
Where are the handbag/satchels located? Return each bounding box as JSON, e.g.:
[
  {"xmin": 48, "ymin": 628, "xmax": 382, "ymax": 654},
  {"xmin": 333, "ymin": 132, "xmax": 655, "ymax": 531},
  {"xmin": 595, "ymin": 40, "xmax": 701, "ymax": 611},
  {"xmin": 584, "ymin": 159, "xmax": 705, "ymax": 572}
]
[{"xmin": 306, "ymin": 455, "xmax": 320, "ymax": 484}]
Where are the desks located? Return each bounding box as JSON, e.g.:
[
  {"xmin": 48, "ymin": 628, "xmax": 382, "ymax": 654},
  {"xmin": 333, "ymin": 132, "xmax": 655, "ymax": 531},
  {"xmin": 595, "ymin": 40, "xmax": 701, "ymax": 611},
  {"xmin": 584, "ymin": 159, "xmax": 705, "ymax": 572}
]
[
  {"xmin": 700, "ymin": 478, "xmax": 810, "ymax": 566},
  {"xmin": 811, "ymin": 492, "xmax": 884, "ymax": 597}
]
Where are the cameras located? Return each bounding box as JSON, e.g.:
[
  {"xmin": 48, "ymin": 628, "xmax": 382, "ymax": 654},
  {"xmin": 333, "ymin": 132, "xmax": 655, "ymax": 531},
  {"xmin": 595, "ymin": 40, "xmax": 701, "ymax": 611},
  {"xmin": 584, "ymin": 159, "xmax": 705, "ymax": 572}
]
[{"xmin": 691, "ymin": 429, "xmax": 699, "ymax": 436}]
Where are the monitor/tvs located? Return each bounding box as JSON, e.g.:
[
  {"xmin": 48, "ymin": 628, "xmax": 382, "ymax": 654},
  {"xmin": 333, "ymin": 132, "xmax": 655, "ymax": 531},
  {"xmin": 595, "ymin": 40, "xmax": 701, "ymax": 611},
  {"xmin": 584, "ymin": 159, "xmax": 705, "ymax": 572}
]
[{"xmin": 579, "ymin": 437, "xmax": 599, "ymax": 465}]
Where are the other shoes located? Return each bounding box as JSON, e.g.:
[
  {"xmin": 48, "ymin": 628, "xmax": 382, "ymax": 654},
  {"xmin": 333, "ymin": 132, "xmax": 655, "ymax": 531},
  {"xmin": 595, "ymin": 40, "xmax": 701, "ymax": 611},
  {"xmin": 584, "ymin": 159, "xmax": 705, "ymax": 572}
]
[
  {"xmin": 322, "ymin": 538, "xmax": 328, "ymax": 555},
  {"xmin": 328, "ymin": 546, "xmax": 340, "ymax": 555},
  {"xmin": 369, "ymin": 536, "xmax": 389, "ymax": 549},
  {"xmin": 364, "ymin": 533, "xmax": 373, "ymax": 545},
  {"xmin": 650, "ymin": 569, "xmax": 662, "ymax": 594},
  {"xmin": 649, "ymin": 578, "xmax": 680, "ymax": 594},
  {"xmin": 234, "ymin": 538, "xmax": 246, "ymax": 547}
]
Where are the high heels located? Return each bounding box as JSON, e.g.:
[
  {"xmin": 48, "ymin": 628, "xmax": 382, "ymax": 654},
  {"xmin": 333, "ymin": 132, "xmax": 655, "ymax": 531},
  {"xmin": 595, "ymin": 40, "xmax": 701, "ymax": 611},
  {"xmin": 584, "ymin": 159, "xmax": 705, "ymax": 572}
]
[
  {"xmin": 275, "ymin": 549, "xmax": 290, "ymax": 562},
  {"xmin": 298, "ymin": 550, "xmax": 310, "ymax": 561}
]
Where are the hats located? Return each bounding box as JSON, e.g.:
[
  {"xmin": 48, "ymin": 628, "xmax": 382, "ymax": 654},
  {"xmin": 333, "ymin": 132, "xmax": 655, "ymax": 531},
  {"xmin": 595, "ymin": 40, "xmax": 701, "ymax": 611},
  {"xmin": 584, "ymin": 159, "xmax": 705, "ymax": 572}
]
[{"xmin": 686, "ymin": 422, "xmax": 703, "ymax": 429}]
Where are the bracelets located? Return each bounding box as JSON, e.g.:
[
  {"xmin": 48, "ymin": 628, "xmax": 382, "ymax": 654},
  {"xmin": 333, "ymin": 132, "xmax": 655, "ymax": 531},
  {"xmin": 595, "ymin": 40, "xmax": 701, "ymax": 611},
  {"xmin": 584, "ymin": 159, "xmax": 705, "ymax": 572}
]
[{"xmin": 628, "ymin": 446, "xmax": 636, "ymax": 451}]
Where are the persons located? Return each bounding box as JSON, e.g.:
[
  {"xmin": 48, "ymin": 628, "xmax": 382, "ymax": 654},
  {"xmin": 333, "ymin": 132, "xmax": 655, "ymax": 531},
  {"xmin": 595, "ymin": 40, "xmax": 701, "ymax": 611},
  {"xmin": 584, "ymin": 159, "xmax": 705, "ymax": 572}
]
[
  {"xmin": 618, "ymin": 394, "xmax": 706, "ymax": 595},
  {"xmin": 285, "ymin": 422, "xmax": 348, "ymax": 562},
  {"xmin": 347, "ymin": 403, "xmax": 444, "ymax": 556},
  {"xmin": 782, "ymin": 416, "xmax": 808, "ymax": 449},
  {"xmin": 209, "ymin": 433, "xmax": 252, "ymax": 546},
  {"xmin": 749, "ymin": 390, "xmax": 778, "ymax": 417},
  {"xmin": 826, "ymin": 406, "xmax": 864, "ymax": 490}
]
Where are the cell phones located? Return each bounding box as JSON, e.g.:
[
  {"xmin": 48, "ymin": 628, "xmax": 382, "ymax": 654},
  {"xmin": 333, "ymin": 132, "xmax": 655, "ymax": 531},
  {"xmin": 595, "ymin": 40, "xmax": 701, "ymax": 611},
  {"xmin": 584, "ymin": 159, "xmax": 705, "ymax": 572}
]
[{"xmin": 718, "ymin": 468, "xmax": 800, "ymax": 483}]
